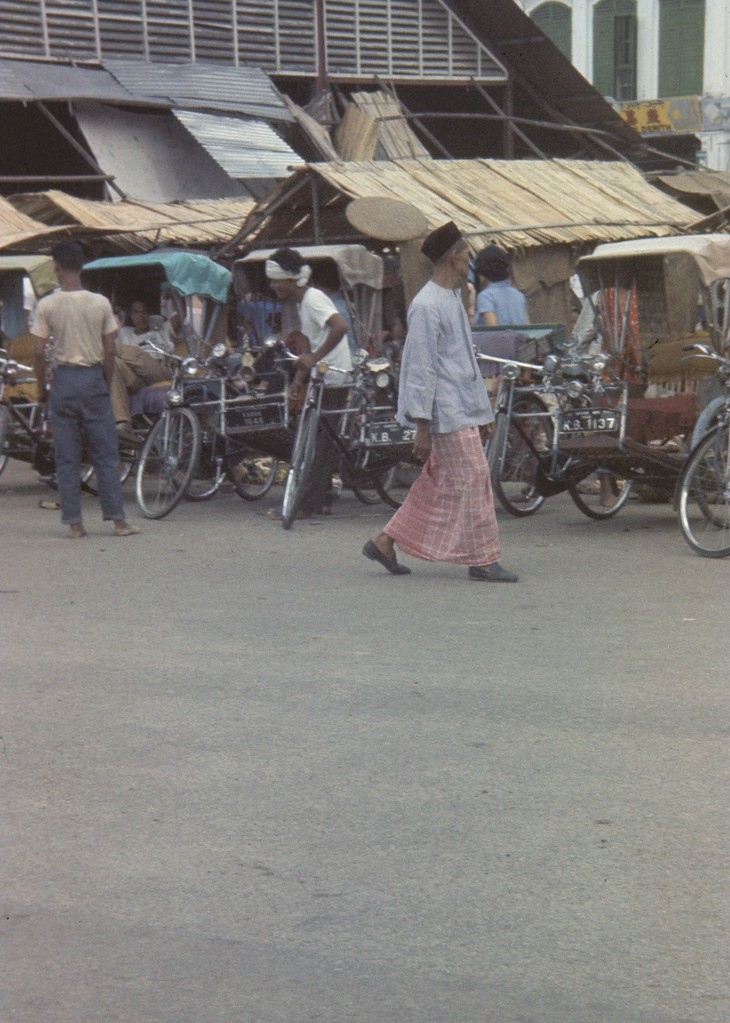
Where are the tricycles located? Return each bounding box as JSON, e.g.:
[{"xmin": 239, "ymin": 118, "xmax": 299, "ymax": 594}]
[{"xmin": 0, "ymin": 233, "xmax": 730, "ymax": 559}]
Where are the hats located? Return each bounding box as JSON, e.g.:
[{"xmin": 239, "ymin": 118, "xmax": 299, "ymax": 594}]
[{"xmin": 420, "ymin": 221, "xmax": 462, "ymax": 263}]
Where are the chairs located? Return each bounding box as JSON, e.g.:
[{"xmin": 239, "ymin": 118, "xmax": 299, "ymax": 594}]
[
  {"xmin": 470, "ymin": 331, "xmax": 528, "ymax": 421},
  {"xmin": 127, "ymin": 338, "xmax": 205, "ymax": 417},
  {"xmin": 603, "ymin": 341, "xmax": 722, "ymax": 438}
]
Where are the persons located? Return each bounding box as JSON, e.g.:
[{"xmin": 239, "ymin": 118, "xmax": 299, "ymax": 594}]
[
  {"xmin": 110, "ymin": 302, "xmax": 170, "ymax": 430},
  {"xmin": 264, "ymin": 251, "xmax": 353, "ymax": 518},
  {"xmin": 571, "ymin": 286, "xmax": 639, "ymax": 386},
  {"xmin": 473, "ymin": 246, "xmax": 531, "ymax": 325},
  {"xmin": 361, "ymin": 222, "xmax": 520, "ymax": 579},
  {"xmin": 30, "ymin": 242, "xmax": 142, "ymax": 538}
]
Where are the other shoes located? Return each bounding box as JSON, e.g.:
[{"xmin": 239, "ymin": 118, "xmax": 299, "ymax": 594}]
[
  {"xmin": 319, "ymin": 504, "xmax": 333, "ymax": 515},
  {"xmin": 115, "ymin": 525, "xmax": 143, "ymax": 537},
  {"xmin": 268, "ymin": 504, "xmax": 313, "ymax": 519},
  {"xmin": 469, "ymin": 561, "xmax": 518, "ymax": 583},
  {"xmin": 116, "ymin": 421, "xmax": 132, "ymax": 433},
  {"xmin": 362, "ymin": 539, "xmax": 411, "ymax": 574},
  {"xmin": 72, "ymin": 528, "xmax": 87, "ymax": 537}
]
